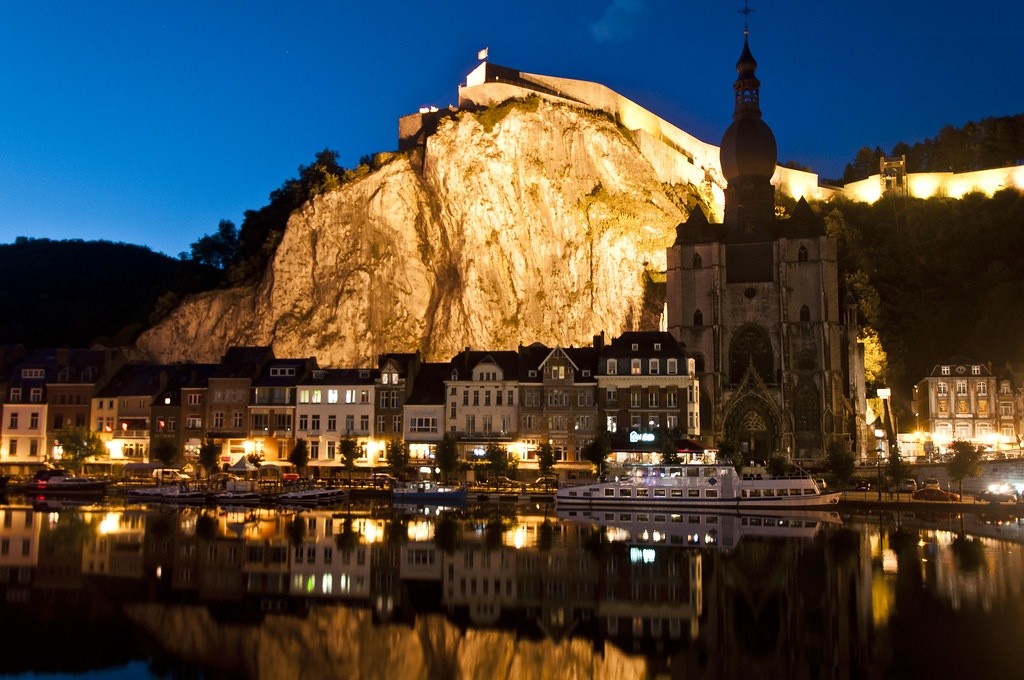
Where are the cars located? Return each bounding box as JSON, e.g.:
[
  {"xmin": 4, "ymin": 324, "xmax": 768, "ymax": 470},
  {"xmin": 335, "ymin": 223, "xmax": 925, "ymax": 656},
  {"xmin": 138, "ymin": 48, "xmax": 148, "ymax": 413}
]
[
  {"xmin": 915, "ymin": 456, "xmax": 927, "ymax": 463},
  {"xmin": 536, "ymin": 477, "xmax": 562, "ymax": 487},
  {"xmin": 912, "ymin": 488, "xmax": 960, "ymax": 501},
  {"xmin": 976, "ymin": 483, "xmax": 1024, "ymax": 502},
  {"xmin": 211, "ymin": 472, "xmax": 242, "ymax": 480},
  {"xmin": 814, "ymin": 478, "xmax": 827, "ymax": 488},
  {"xmin": 487, "ymin": 476, "xmax": 512, "ymax": 485}
]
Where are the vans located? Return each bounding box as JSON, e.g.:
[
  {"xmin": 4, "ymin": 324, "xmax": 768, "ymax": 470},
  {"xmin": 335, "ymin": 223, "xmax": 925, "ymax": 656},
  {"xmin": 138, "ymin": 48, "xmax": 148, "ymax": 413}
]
[{"xmin": 152, "ymin": 468, "xmax": 190, "ymax": 480}]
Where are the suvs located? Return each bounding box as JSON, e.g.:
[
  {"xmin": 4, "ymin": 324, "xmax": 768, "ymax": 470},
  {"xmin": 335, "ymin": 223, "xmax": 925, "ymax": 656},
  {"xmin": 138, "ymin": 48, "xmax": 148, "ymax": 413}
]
[
  {"xmin": 900, "ymin": 478, "xmax": 918, "ymax": 492},
  {"xmin": 370, "ymin": 474, "xmax": 397, "ymax": 483},
  {"xmin": 856, "ymin": 480, "xmax": 871, "ymax": 491},
  {"xmin": 923, "ymin": 478, "xmax": 941, "ymax": 491}
]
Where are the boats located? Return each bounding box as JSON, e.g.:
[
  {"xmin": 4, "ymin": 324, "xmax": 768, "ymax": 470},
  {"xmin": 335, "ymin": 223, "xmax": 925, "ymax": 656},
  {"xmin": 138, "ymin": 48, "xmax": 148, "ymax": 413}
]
[
  {"xmin": 271, "ymin": 481, "xmax": 348, "ymax": 499},
  {"xmin": 212, "ymin": 487, "xmax": 260, "ymax": 501},
  {"xmin": 125, "ymin": 475, "xmax": 205, "ymax": 499},
  {"xmin": 557, "ymin": 438, "xmax": 842, "ymax": 508},
  {"xmin": 557, "ymin": 504, "xmax": 841, "ymax": 550},
  {"xmin": 392, "ymin": 480, "xmax": 468, "ymax": 499},
  {"xmin": 19, "ymin": 470, "xmax": 108, "ymax": 492}
]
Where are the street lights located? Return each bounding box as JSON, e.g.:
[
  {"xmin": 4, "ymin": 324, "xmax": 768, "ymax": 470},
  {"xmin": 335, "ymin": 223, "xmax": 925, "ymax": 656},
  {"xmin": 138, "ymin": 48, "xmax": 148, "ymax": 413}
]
[{"xmin": 875, "ymin": 449, "xmax": 886, "ymax": 500}]
